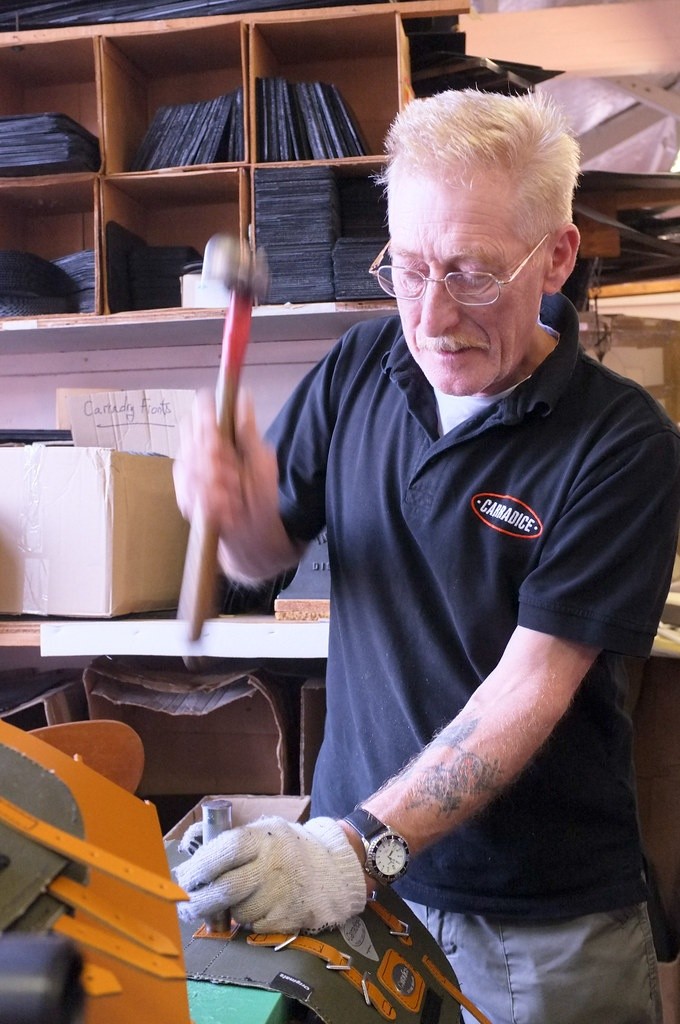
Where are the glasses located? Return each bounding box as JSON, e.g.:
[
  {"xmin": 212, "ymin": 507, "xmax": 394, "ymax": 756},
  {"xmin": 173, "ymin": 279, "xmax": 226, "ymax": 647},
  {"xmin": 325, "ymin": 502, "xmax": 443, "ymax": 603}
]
[{"xmin": 369, "ymin": 232, "xmax": 554, "ymax": 306}]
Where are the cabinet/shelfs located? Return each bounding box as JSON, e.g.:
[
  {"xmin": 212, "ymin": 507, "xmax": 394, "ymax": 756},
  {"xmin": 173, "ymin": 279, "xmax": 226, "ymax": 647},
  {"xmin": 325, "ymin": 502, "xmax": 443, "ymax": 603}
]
[{"xmin": 0, "ymin": 0, "xmax": 476, "ymax": 331}]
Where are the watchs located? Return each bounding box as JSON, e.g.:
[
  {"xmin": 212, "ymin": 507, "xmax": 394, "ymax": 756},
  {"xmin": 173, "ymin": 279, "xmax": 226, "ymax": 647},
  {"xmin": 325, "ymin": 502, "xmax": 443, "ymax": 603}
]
[{"xmin": 342, "ymin": 807, "xmax": 412, "ymax": 888}]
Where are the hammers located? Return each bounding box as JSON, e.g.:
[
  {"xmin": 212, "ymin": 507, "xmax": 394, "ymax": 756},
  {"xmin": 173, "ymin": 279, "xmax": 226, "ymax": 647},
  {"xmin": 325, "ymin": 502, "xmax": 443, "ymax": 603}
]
[{"xmin": 177, "ymin": 236, "xmax": 270, "ymax": 643}]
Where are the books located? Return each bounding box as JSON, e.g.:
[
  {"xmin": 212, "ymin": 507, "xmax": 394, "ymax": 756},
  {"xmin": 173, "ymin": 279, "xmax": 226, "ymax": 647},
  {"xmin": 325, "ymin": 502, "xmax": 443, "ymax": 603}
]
[{"xmin": 570, "ymin": 203, "xmax": 680, "ymax": 309}]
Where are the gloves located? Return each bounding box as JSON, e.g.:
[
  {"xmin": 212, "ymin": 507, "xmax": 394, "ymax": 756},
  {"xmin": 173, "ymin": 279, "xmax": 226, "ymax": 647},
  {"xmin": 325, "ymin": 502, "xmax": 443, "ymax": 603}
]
[{"xmin": 172, "ymin": 817, "xmax": 367, "ymax": 938}]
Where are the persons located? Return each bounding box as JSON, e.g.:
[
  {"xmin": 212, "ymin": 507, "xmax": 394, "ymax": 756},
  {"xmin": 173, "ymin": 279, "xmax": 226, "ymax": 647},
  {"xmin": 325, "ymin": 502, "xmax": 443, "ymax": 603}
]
[{"xmin": 168, "ymin": 88, "xmax": 680, "ymax": 1024}]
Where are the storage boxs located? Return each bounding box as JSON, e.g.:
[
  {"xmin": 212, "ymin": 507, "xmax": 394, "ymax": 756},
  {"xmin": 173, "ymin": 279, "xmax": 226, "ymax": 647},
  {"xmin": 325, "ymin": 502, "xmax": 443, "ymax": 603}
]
[
  {"xmin": 162, "ymin": 793, "xmax": 312, "ymax": 844},
  {"xmin": 0, "ymin": 439, "xmax": 194, "ymax": 620}
]
